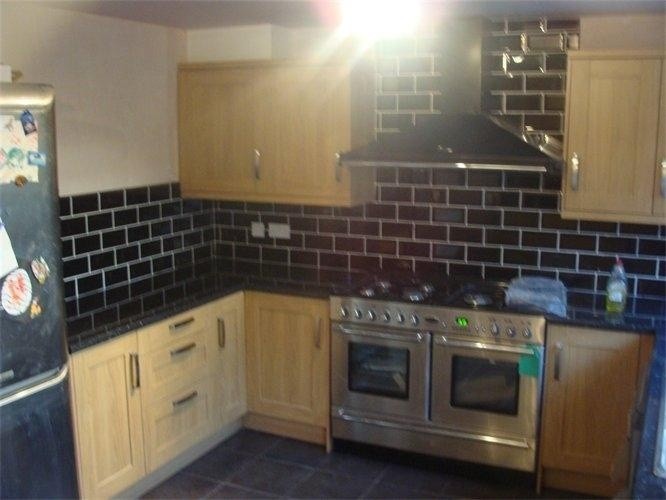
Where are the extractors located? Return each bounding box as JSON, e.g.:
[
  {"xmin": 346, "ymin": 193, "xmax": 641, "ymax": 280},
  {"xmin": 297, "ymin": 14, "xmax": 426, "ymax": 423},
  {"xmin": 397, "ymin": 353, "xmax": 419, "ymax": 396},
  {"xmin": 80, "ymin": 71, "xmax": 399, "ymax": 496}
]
[{"xmin": 337, "ymin": 15, "xmax": 564, "ymax": 174}]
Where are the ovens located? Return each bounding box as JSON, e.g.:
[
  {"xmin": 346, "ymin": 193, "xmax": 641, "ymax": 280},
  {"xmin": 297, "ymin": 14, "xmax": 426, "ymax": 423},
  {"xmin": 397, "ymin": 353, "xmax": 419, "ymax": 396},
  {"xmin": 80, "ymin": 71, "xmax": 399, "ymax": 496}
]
[{"xmin": 326, "ymin": 294, "xmax": 547, "ymax": 478}]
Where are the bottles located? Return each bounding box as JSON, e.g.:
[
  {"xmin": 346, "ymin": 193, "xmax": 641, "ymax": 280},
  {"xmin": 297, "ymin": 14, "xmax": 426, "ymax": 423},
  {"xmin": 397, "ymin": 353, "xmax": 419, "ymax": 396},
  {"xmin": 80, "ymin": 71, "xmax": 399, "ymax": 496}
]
[{"xmin": 603, "ymin": 255, "xmax": 629, "ymax": 314}]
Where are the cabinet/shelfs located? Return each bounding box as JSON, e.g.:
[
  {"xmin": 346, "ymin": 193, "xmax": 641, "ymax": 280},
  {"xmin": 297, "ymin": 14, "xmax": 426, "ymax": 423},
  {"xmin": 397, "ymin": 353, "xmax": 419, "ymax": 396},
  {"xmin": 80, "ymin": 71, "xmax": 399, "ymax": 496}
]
[
  {"xmin": 533, "ymin": 321, "xmax": 652, "ymax": 499},
  {"xmin": 67, "ymin": 289, "xmax": 244, "ymax": 500},
  {"xmin": 177, "ymin": 60, "xmax": 376, "ymax": 208},
  {"xmin": 559, "ymin": 47, "xmax": 666, "ymax": 226},
  {"xmin": 245, "ymin": 290, "xmax": 333, "ymax": 454}
]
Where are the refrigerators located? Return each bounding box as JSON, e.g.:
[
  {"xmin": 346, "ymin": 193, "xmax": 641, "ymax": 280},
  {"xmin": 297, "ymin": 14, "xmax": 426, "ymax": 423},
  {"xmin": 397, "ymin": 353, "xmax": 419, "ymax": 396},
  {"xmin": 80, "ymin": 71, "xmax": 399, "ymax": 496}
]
[{"xmin": 0, "ymin": 79, "xmax": 82, "ymax": 500}]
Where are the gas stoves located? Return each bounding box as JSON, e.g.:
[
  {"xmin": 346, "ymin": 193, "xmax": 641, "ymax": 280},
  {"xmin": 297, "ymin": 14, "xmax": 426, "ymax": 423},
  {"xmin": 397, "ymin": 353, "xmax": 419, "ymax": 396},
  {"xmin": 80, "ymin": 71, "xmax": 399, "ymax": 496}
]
[{"xmin": 334, "ymin": 269, "xmax": 507, "ymax": 310}]
